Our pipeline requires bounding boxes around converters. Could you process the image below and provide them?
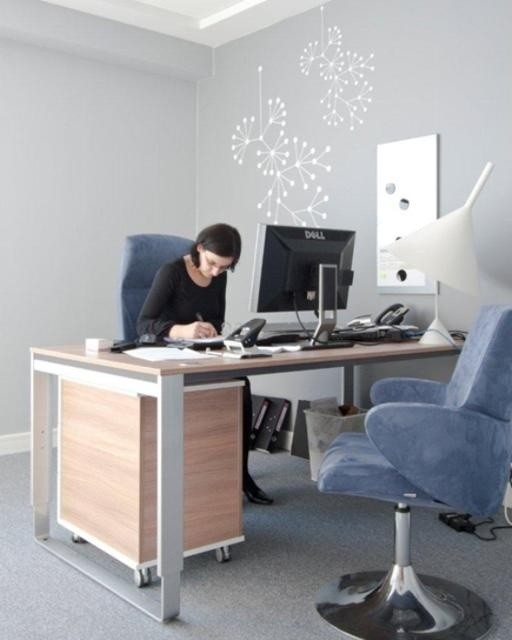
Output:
[438,512,476,531]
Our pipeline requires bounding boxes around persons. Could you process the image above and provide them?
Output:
[136,224,274,504]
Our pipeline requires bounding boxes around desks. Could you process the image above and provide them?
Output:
[28,331,466,623]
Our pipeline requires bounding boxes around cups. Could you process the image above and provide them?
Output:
[138,333,156,346]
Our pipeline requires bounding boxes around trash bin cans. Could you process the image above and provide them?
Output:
[303,404,370,482]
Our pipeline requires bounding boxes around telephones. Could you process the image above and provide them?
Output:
[375,304,410,326]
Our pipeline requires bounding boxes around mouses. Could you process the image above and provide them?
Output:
[140,333,158,346]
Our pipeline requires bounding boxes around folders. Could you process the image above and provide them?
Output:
[248,395,271,450]
[255,397,290,455]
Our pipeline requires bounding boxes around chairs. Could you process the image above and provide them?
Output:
[117,233,200,346]
[315,306,512,640]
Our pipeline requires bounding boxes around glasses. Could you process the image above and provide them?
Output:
[204,249,233,272]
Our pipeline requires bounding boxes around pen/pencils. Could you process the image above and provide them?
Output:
[196,312,204,322]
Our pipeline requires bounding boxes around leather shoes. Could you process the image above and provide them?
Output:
[243,475,274,506]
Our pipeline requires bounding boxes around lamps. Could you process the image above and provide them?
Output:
[379,163,497,349]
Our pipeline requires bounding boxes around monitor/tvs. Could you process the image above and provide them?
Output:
[249,222,357,352]
